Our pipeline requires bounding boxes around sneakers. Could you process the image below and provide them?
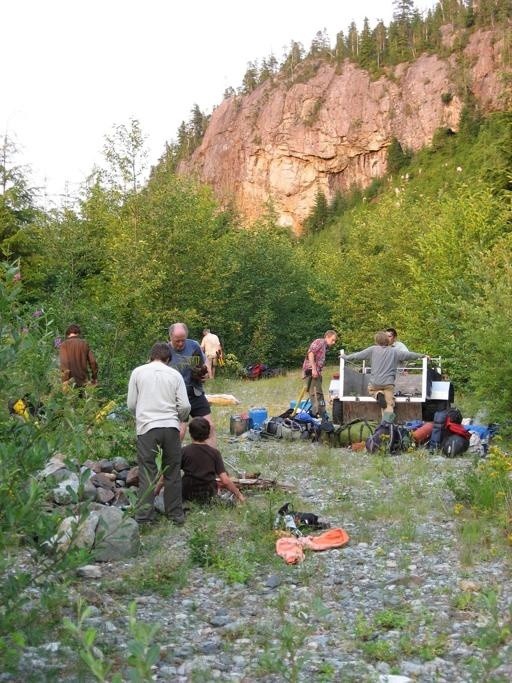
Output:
[376,391,387,408]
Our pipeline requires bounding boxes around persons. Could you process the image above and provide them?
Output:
[62,325,98,398]
[200,328,222,380]
[302,330,338,418]
[328,371,343,424]
[126,344,191,528]
[386,329,410,374]
[154,416,245,510]
[339,331,431,419]
[166,322,216,448]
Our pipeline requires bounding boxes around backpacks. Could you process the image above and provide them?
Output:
[337,416,378,446]
[403,406,472,457]
[265,409,337,445]
[366,421,401,455]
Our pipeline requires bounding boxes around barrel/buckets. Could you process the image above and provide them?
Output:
[249,406,267,429]
